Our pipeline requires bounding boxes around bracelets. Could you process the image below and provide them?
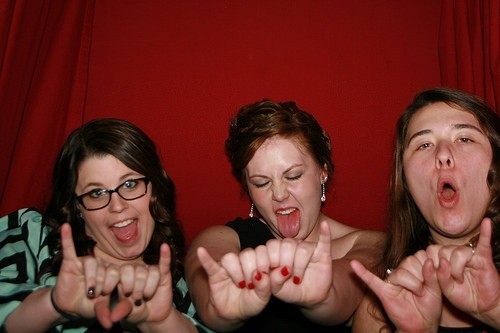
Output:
[50,285,88,323]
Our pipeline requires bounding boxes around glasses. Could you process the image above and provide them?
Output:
[73,173,154,211]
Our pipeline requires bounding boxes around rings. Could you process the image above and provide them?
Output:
[386,268,395,285]
[464,242,475,258]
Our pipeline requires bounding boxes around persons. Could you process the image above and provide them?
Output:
[349,87,500,333]
[183,98,389,333]
[0,118,211,333]
